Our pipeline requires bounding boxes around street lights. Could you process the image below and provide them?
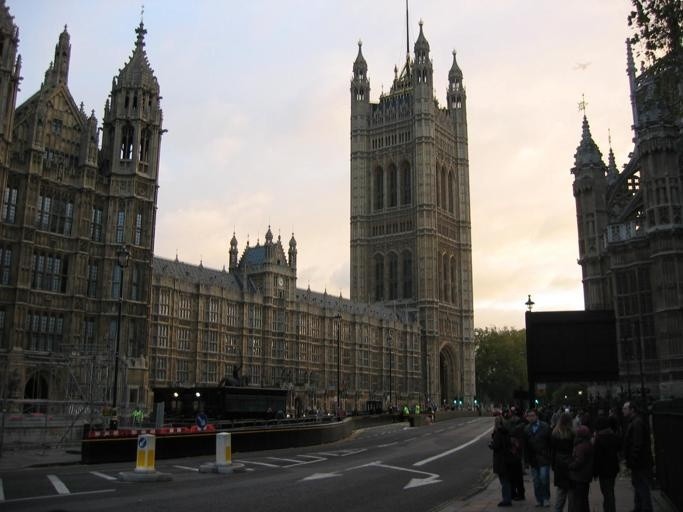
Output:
[334,313,342,413]
[387,333,394,406]
[110,244,134,430]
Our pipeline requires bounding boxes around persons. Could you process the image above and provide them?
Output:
[196,410,208,431]
[132,407,144,426]
[486,400,656,510]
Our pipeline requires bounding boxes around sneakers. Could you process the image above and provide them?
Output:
[497,493,551,508]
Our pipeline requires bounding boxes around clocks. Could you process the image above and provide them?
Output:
[277,276,284,287]
